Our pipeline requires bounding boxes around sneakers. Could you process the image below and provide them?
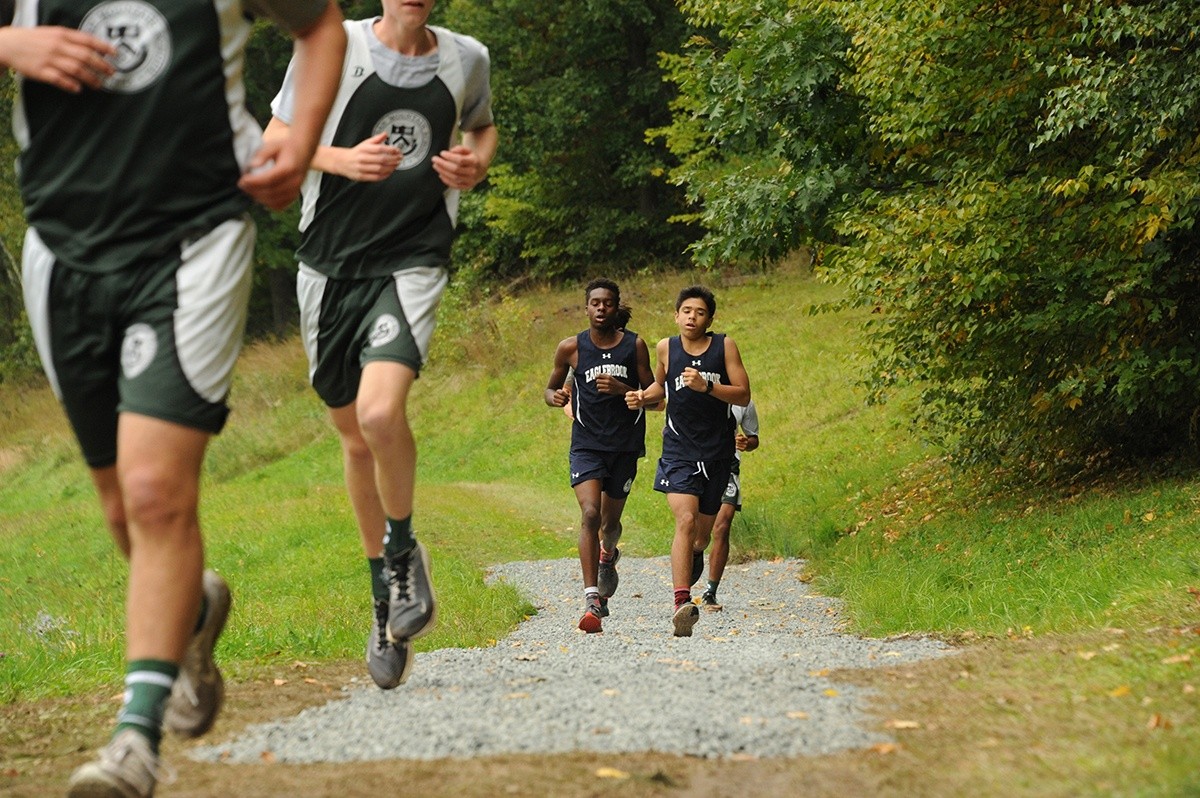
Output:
[577,604,603,633]
[699,592,722,612]
[381,540,437,642]
[68,727,158,796]
[162,568,231,739]
[692,549,703,586]
[672,601,699,637]
[597,547,621,598]
[367,595,414,689]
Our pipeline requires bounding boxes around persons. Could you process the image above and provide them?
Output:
[0,0,350,798]
[625,287,751,638]
[542,280,660,634]
[561,303,667,618]
[701,398,760,611]
[260,1,500,690]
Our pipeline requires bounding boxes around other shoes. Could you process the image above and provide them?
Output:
[600,596,610,617]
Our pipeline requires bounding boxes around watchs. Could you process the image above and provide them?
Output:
[704,380,713,394]
